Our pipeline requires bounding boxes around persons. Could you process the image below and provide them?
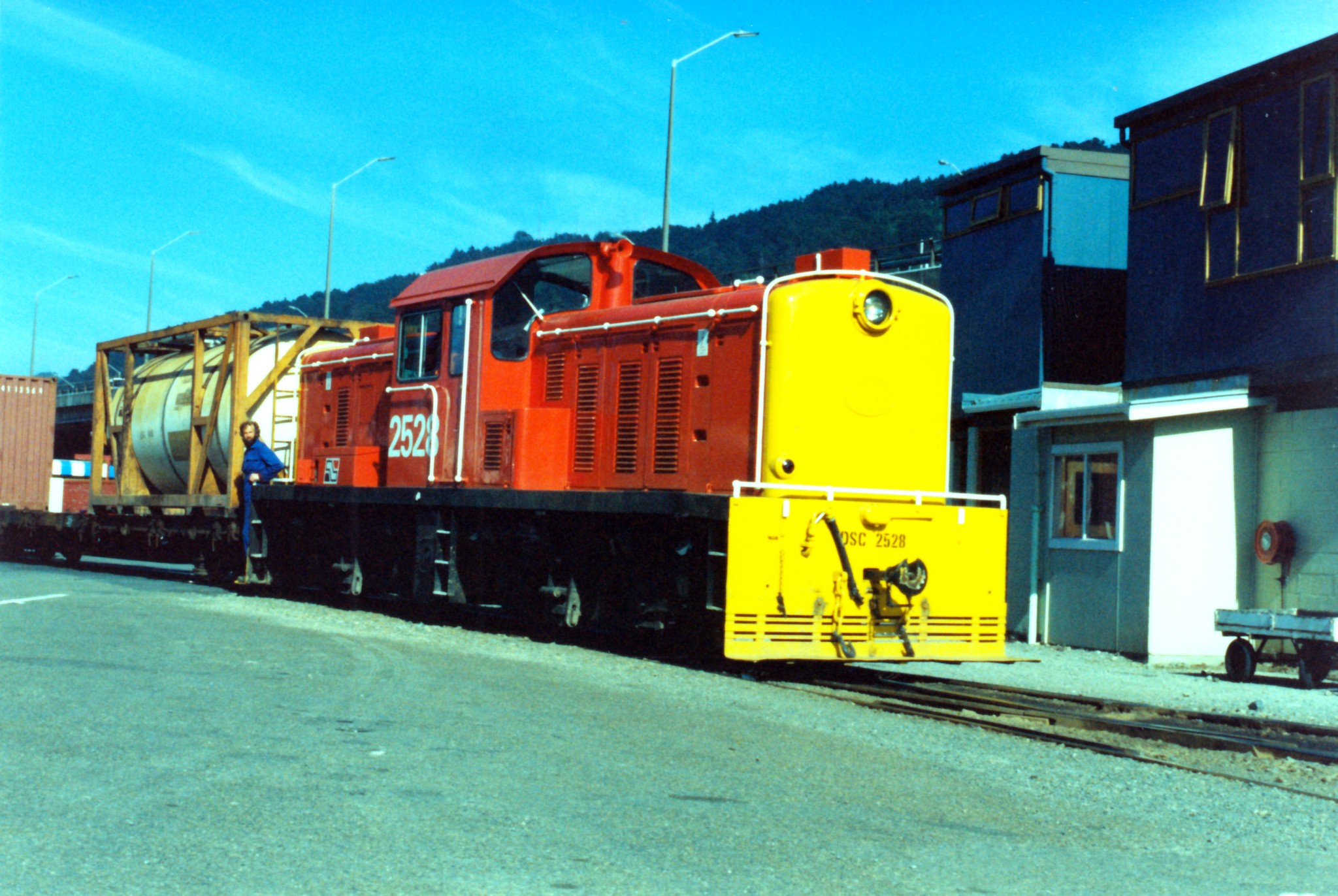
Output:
[235,421,285,584]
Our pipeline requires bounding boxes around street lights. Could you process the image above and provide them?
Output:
[325,153,395,321]
[664,32,766,254]
[149,233,195,330]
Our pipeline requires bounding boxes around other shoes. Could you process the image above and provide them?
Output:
[237,575,245,583]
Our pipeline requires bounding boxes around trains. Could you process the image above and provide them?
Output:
[0,238,1014,666]
